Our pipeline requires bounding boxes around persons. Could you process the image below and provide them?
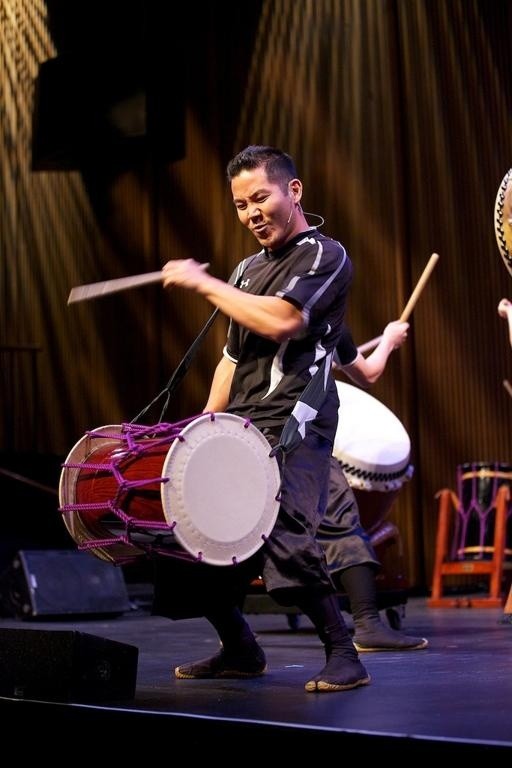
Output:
[313,316,429,655]
[162,142,372,692]
[497,298,512,350]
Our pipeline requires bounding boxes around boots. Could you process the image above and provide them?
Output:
[352,598,428,651]
[305,621,372,691]
[175,623,267,677]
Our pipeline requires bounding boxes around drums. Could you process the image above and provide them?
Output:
[454,460,510,560]
[332,380,413,537]
[57,411,282,569]
[495,165,512,275]
